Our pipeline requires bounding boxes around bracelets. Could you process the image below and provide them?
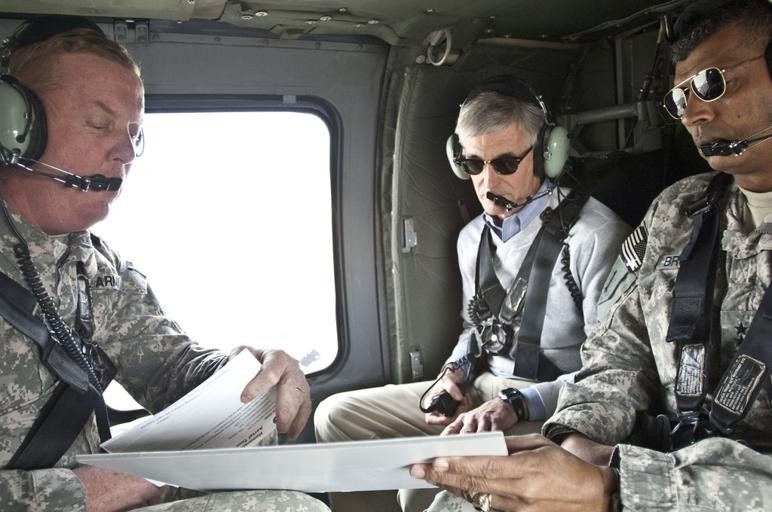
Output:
[607,462,623,512]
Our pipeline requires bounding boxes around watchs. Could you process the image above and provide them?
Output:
[498,386,525,423]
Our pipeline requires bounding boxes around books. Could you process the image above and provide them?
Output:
[97,347,279,489]
[73,428,510,495]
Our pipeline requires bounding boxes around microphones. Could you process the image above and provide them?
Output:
[699,133,771,157]
[16,160,122,193]
[485,181,558,211]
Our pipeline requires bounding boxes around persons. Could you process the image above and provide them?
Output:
[310,73,634,512]
[1,14,332,512]
[408,2,772,512]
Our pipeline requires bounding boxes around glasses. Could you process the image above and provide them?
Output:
[662,55,762,120]
[461,145,533,175]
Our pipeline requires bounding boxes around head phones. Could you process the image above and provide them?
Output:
[0,15,105,169]
[446,74,570,179]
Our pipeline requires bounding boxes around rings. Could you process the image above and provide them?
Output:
[295,386,305,393]
[471,492,492,512]
